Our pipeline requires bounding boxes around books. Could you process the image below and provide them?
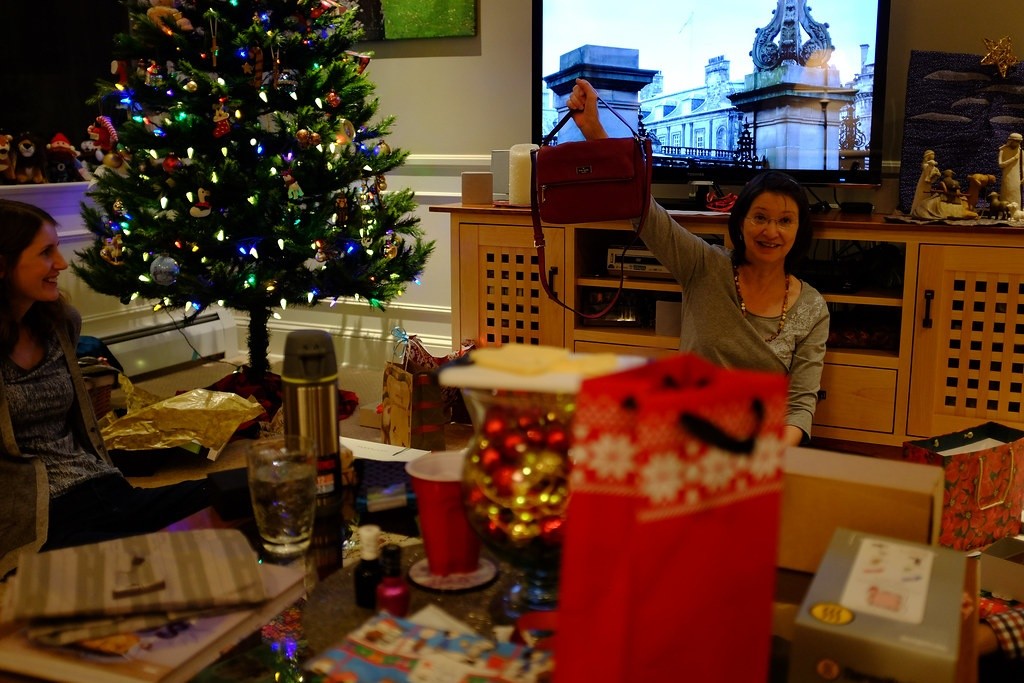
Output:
[0,529,305,683]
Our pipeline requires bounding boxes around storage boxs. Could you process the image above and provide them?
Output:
[788,521,981,678]
[755,446,955,576]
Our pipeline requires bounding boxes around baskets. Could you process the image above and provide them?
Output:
[85,375,114,420]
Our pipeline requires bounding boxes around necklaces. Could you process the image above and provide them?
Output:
[733,266,790,343]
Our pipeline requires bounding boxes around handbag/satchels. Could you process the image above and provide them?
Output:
[902,420,1024,552]
[531,97,652,318]
[381,338,447,454]
[552,351,787,683]
[405,334,478,413]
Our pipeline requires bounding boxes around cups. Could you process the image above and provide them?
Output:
[246,436,316,553]
[405,449,480,571]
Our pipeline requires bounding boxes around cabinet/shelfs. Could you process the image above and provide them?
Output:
[426,204,1018,443]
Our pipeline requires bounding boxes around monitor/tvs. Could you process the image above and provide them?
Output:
[532,0,891,211]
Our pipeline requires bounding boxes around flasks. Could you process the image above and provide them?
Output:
[282,328,340,470]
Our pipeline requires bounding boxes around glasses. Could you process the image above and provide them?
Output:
[745,214,800,229]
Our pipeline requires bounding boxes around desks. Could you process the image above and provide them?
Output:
[0,437,1024,683]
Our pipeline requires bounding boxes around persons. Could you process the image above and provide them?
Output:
[0,198,139,552]
[566,79,830,448]
[910,151,934,216]
[968,175,996,205]
[998,133,1024,209]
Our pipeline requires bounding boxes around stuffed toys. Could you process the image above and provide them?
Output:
[0,133,82,184]
